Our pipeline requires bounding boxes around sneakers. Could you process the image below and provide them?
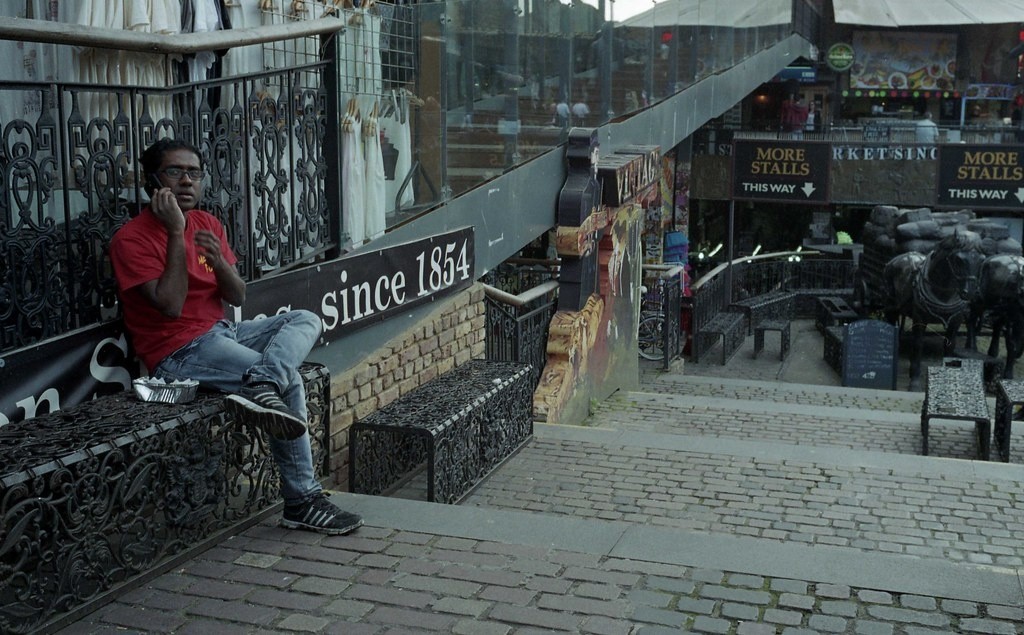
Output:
[224,386,308,441]
[282,491,365,535]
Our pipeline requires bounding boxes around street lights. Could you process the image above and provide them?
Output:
[566,2,576,129]
[438,13,456,196]
[505,6,523,167]
[607,0,617,119]
[650,0,659,103]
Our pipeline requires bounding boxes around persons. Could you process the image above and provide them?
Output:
[778,78,809,139]
[551,93,591,127]
[587,20,629,117]
[109,137,364,534]
[915,111,939,143]
[802,100,823,134]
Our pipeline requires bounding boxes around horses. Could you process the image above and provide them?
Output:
[881,228,1023,377]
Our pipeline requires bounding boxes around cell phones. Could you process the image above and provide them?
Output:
[144,174,162,198]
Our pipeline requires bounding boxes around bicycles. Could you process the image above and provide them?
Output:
[639,286,681,361]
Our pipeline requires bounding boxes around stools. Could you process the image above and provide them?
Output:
[824,326,846,374]
[994,379,1024,463]
[753,318,791,360]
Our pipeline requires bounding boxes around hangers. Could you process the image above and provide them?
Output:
[223,0,389,31]
[228,75,425,138]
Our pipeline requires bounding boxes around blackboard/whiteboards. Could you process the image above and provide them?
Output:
[841,318,900,390]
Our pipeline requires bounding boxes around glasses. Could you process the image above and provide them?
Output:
[156,168,206,182]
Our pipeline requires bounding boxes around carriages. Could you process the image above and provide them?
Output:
[854,205,1024,390]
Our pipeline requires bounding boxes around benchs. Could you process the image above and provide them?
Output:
[920,366,991,460]
[350,358,535,506]
[0,361,334,635]
[728,290,797,337]
[797,287,855,318]
[695,312,746,365]
